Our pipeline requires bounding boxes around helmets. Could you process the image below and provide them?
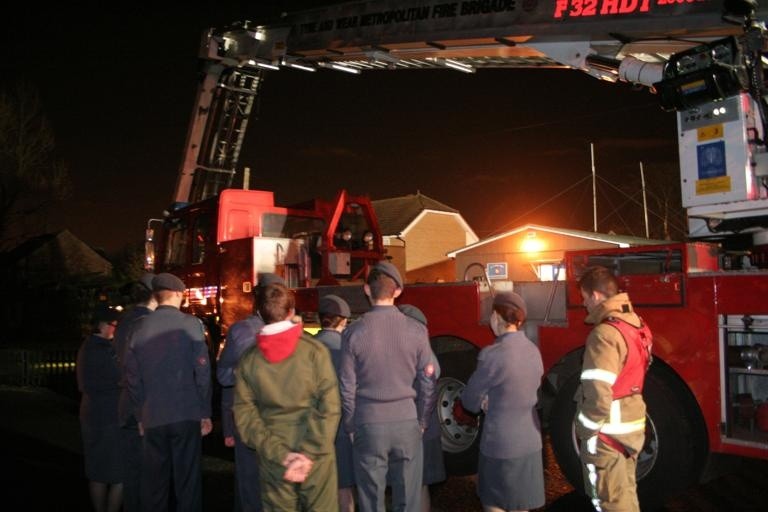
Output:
[398,303,427,323]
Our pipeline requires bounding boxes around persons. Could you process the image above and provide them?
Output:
[387,304,445,510]
[218,272,287,510]
[572,264,654,512]
[126,273,213,511]
[339,262,436,510]
[76,306,129,509]
[113,274,157,509]
[233,283,342,510]
[459,288,549,512]
[312,294,356,510]
[334,220,375,278]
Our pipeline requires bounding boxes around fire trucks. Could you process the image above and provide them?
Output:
[133,9,766,510]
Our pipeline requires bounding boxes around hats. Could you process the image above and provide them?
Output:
[317,294,351,317]
[252,272,286,293]
[493,290,527,312]
[140,273,186,291]
[370,261,403,287]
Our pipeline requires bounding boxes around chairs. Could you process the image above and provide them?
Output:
[736,393,762,434]
